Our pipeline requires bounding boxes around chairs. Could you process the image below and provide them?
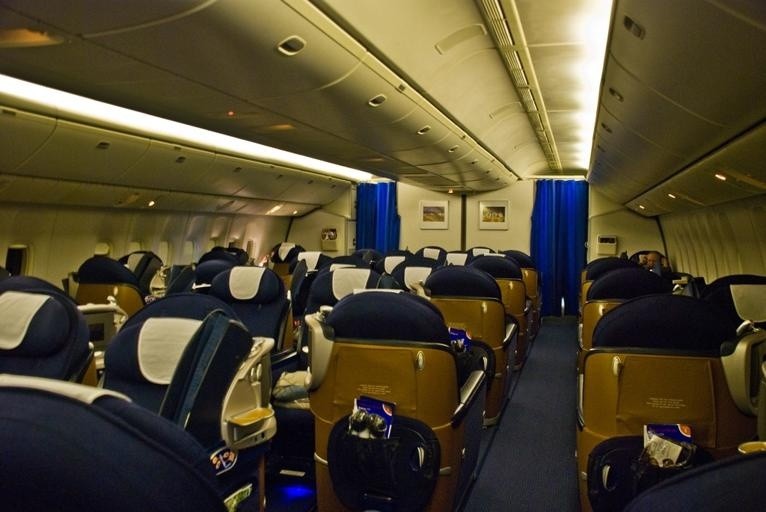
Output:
[568,243,766,511]
[0,244,547,511]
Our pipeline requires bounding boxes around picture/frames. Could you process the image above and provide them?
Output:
[418,199,450,230]
[479,199,510,231]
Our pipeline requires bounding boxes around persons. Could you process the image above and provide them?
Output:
[638,250,669,269]
[257,244,280,266]
